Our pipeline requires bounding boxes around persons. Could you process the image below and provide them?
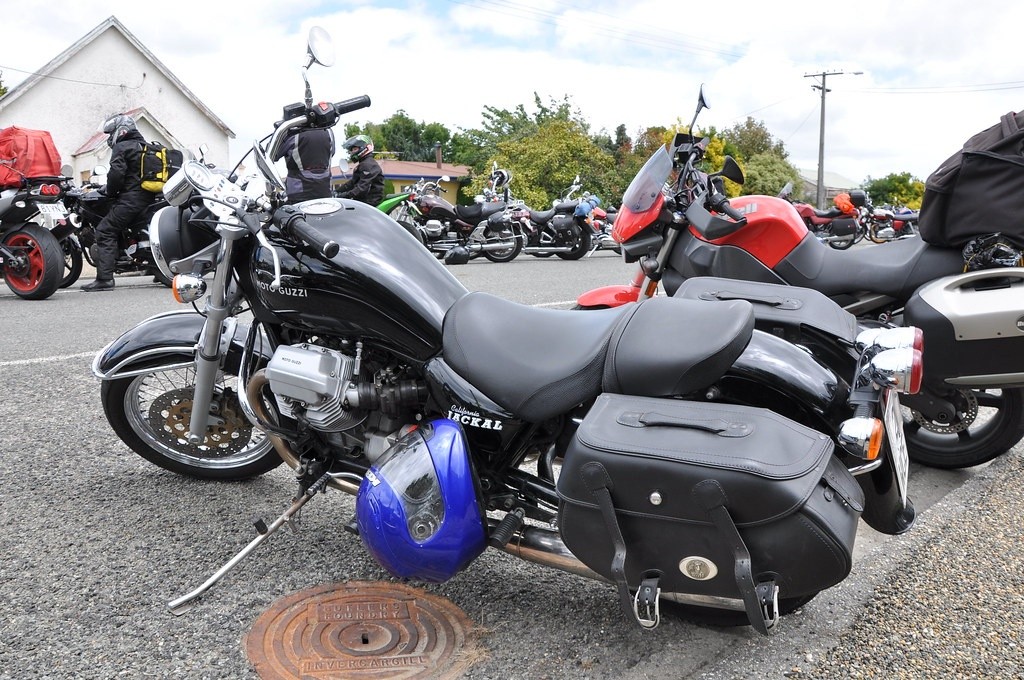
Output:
[332,134,387,207]
[272,127,335,205]
[80,112,155,293]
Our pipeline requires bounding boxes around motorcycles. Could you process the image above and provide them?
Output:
[0,175,74,300]
[90,22,925,637]
[474,161,598,260]
[570,82,1024,470]
[775,181,867,251]
[403,174,534,264]
[529,174,622,258]
[57,164,211,290]
[870,209,922,243]
[374,192,424,246]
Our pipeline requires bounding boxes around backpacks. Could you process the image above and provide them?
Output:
[917,110,1024,248]
[129,138,184,193]
[0,127,61,187]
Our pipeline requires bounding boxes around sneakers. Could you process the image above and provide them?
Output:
[80,278,115,292]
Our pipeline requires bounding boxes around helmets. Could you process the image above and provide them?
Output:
[104,113,137,148]
[490,169,512,189]
[355,418,489,585]
[342,134,374,163]
